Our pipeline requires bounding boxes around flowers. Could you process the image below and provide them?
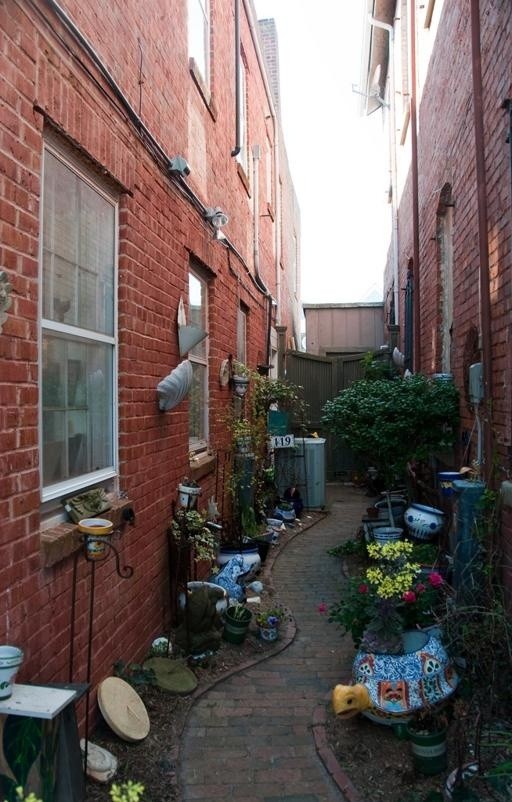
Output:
[317,539,445,649]
[253,600,292,627]
[364,538,423,655]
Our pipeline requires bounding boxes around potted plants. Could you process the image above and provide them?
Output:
[231,419,255,454]
[406,700,449,775]
[222,600,252,645]
[232,359,259,396]
[239,504,274,548]
[273,502,296,523]
[177,477,201,509]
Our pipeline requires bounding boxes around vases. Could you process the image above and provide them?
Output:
[373,471,465,544]
[333,630,460,740]
[256,621,280,642]
[390,603,418,632]
[216,544,262,582]
[78,518,113,560]
[0,644,24,700]
